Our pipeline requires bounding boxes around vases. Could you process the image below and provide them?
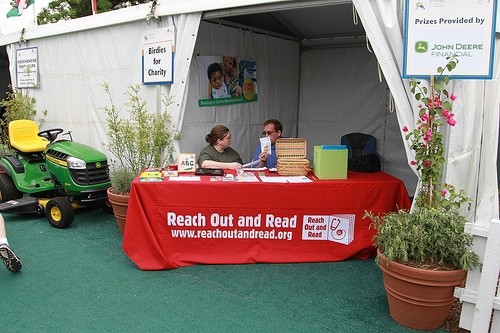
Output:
[374,244,467,331]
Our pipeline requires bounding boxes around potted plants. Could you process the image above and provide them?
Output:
[94,78,185,236]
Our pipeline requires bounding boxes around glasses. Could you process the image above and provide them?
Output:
[263,130,276,135]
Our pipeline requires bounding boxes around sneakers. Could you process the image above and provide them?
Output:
[0,243,23,272]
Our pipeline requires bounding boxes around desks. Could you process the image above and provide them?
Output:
[122,168,414,272]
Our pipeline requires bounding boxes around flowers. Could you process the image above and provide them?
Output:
[360,53,485,274]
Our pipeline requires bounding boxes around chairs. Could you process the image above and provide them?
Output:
[8,119,50,154]
[340,132,378,160]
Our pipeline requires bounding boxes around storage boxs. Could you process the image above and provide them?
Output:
[274,136,310,176]
[313,145,348,180]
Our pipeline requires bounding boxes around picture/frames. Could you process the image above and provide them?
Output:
[141,24,175,85]
[401,0,497,81]
[15,46,41,89]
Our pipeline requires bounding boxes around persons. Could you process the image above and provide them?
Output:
[197,123,248,172]
[0,214,23,273]
[207,62,233,98]
[251,118,290,170]
